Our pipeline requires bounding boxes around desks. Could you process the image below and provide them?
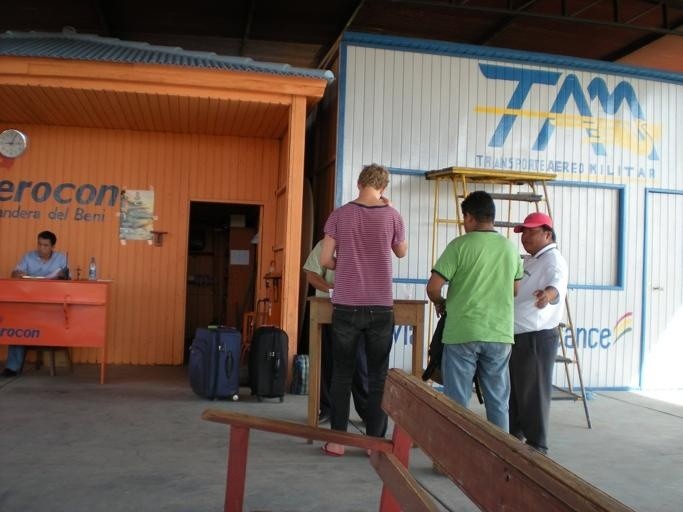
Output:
[0,276,112,386]
[303,296,429,445]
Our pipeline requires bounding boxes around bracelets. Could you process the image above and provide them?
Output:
[435,298,444,307]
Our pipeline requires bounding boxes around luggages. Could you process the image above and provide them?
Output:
[248,299,288,402]
[188,321,242,402]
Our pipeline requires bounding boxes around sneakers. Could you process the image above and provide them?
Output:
[319,411,331,424]
[3,367,17,378]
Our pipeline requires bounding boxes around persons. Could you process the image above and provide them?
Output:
[319,163,407,456]
[509,212,569,457]
[0,231,68,379]
[301,238,338,425]
[425,191,525,476]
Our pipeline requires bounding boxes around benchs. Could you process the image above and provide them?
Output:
[198,367,632,511]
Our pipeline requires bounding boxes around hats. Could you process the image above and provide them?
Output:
[514,213,553,233]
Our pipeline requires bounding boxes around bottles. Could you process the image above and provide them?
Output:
[88,257,96,281]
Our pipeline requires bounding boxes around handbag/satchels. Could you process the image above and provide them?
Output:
[422,311,447,386]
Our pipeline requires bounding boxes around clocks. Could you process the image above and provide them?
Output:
[0,129,27,159]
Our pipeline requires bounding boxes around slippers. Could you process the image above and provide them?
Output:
[321,443,344,456]
